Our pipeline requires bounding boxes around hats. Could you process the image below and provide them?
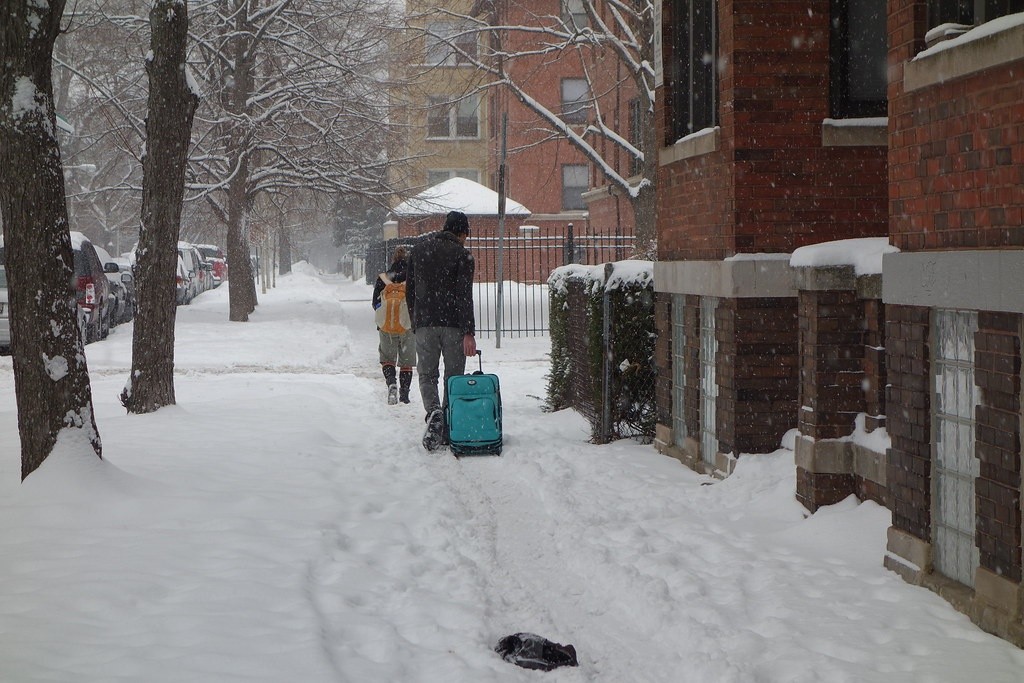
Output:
[444,211,469,235]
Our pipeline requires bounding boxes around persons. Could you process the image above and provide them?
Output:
[405,211,476,452]
[372,246,416,406]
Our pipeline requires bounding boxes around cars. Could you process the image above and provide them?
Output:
[0,231,260,356]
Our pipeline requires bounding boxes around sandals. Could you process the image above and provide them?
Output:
[387,384,399,405]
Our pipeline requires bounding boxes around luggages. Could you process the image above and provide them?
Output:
[444,349,502,457]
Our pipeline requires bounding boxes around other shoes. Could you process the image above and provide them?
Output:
[422,410,445,452]
[399,386,410,404]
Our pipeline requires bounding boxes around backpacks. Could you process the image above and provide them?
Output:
[374,272,412,335]
